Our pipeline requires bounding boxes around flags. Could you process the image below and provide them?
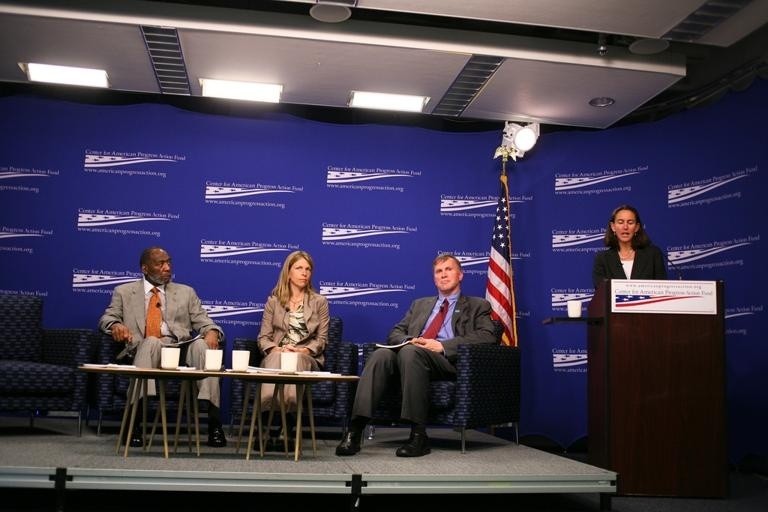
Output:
[486,180,517,348]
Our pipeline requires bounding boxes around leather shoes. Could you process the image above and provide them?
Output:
[275,438,295,452]
[396,431,431,457]
[336,431,362,456]
[253,437,273,452]
[207,426,227,447]
[122,425,142,447]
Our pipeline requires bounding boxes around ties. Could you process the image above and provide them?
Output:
[145,286,162,338]
[422,298,449,340]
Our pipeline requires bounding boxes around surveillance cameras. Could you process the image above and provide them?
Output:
[597,45,609,57]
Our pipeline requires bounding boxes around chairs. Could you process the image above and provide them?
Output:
[226,313,362,445]
[361,315,528,456]
[0,285,95,437]
[93,325,224,442]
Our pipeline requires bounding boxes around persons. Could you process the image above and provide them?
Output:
[593,206,668,288]
[97,247,227,448]
[335,254,496,457]
[255,249,331,454]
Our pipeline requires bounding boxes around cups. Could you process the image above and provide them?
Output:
[230,349,251,371]
[567,301,582,318]
[204,348,224,370]
[281,350,299,372]
[160,346,182,369]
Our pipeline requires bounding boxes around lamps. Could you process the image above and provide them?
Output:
[502,118,541,162]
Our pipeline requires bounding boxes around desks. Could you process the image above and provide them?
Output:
[76,362,222,460]
[223,367,360,462]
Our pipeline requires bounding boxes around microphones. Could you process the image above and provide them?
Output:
[648,242,687,280]
